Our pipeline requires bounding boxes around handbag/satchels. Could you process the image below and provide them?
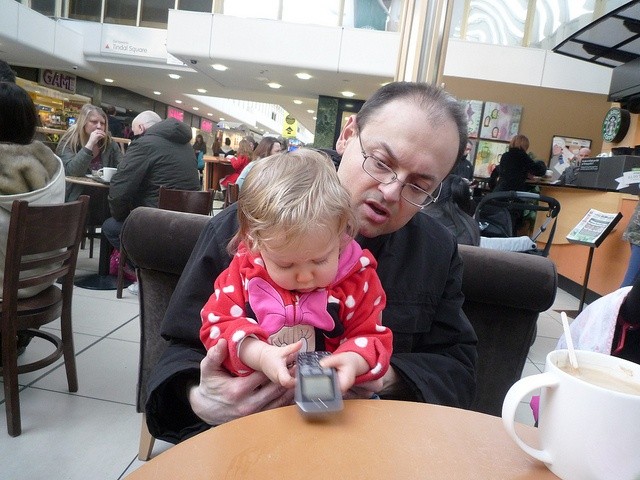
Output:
[197,150,205,169]
[110,248,137,282]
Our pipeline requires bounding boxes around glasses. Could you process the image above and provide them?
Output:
[357,125,442,208]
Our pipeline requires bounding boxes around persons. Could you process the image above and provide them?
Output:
[447,138,475,180]
[0,78,68,372]
[55,100,125,201]
[210,135,220,156]
[217,137,234,157]
[618,201,639,287]
[107,106,123,138]
[556,146,593,185]
[232,136,280,191]
[193,134,207,156]
[195,144,396,391]
[139,80,479,449]
[101,109,201,270]
[489,132,547,234]
[219,139,249,190]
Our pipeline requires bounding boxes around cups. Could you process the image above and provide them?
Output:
[501,349,640,480]
[219,153,225,159]
[97,166,117,183]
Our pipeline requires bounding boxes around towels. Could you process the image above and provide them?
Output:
[554,285,632,356]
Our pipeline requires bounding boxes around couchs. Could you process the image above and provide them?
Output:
[120,207,558,461]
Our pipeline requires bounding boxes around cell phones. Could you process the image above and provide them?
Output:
[293,350,343,415]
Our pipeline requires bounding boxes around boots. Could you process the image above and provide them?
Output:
[222,189,228,208]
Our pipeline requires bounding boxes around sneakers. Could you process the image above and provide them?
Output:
[128,281,139,296]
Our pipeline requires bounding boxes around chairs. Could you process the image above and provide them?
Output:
[116,185,214,300]
[472,191,560,258]
[224,182,239,206]
[0,195,90,437]
[81,224,103,258]
